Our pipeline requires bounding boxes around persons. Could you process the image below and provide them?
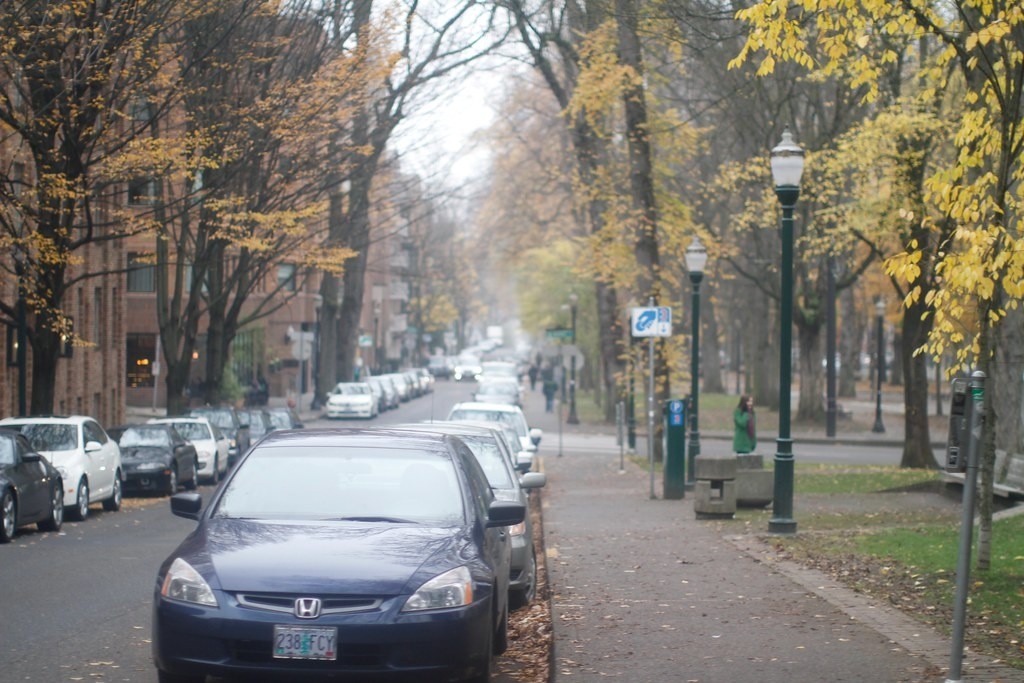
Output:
[536,351,543,367]
[541,364,553,393]
[529,363,539,389]
[196,377,270,407]
[734,394,757,454]
[542,376,559,413]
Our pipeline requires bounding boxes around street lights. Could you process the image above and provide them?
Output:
[767,120,806,534]
[681,235,718,477]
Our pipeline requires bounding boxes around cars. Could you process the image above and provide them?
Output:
[104,421,199,497]
[143,416,230,484]
[2,413,127,521]
[155,422,528,683]
[0,427,66,543]
[368,321,548,607]
[186,402,304,478]
[325,381,379,419]
